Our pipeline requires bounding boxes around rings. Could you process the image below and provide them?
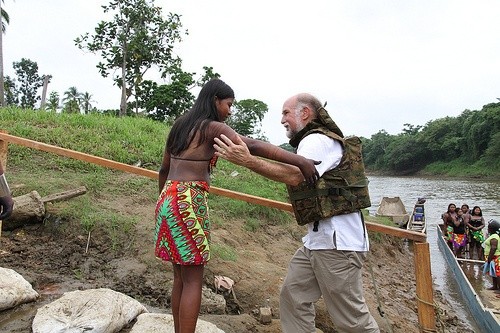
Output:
[223,151,225,154]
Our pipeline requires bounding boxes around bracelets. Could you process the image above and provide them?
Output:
[0,173,11,199]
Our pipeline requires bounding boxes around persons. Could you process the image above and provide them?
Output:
[154,78,322,333]
[483,218,500,300]
[441,203,486,265]
[0,159,14,220]
[211,91,381,333]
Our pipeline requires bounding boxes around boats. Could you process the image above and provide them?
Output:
[375,196,410,228]
[436,220,500,333]
[406,197,426,234]
